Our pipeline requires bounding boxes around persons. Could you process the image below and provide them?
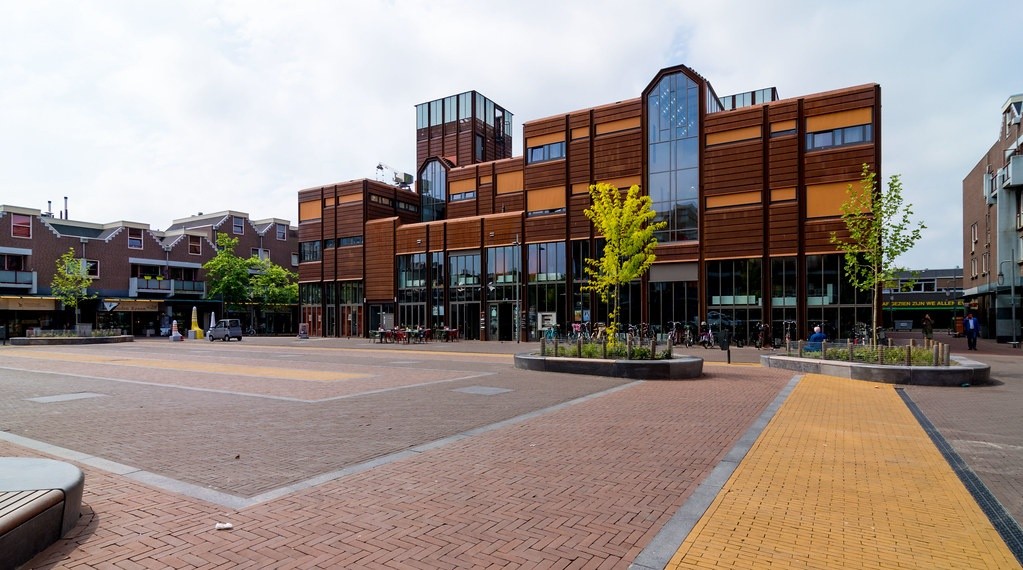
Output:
[922,314,934,340]
[378,322,449,343]
[962,312,981,351]
[810,327,826,342]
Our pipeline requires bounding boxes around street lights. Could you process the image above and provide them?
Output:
[945,269,957,334]
[889,288,895,331]
[997,249,1017,342]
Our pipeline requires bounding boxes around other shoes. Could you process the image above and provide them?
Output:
[968,346,977,350]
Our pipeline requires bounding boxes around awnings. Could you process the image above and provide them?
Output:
[0,294,64,310]
[97,297,223,312]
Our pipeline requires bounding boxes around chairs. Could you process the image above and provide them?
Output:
[368,327,459,343]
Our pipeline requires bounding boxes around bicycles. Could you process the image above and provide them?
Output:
[683,324,694,349]
[567,321,660,345]
[545,323,560,344]
[756,322,769,349]
[782,321,797,347]
[667,321,682,346]
[701,321,716,348]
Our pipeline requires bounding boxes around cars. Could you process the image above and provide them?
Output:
[208,318,242,342]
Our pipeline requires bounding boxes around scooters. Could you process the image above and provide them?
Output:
[812,322,825,336]
[852,322,889,346]
